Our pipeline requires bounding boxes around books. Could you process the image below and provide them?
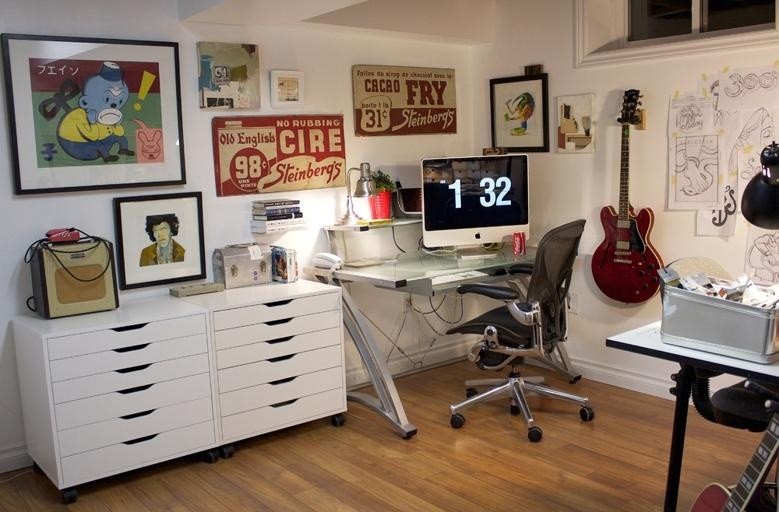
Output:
[250,199,306,233]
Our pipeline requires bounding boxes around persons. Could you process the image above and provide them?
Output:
[140,214,185,267]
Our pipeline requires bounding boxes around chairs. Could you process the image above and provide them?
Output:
[449,219,595,443]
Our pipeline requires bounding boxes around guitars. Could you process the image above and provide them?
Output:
[591,89,664,303]
[689,407,779,511]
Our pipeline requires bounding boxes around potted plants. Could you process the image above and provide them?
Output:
[368,169,392,220]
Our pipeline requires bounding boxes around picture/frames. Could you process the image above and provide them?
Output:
[270,70,304,109]
[196,41,261,111]
[489,72,550,154]
[112,191,207,290]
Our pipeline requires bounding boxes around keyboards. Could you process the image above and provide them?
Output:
[432,270,488,287]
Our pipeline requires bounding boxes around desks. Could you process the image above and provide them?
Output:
[604,316,779,512]
[303,218,538,437]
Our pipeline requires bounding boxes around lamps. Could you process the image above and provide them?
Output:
[333,162,378,226]
[741,142,779,230]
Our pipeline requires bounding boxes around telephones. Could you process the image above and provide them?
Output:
[312,253,343,285]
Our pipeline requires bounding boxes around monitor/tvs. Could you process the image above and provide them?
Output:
[421,154,530,261]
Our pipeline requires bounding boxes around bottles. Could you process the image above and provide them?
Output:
[514,231,526,256]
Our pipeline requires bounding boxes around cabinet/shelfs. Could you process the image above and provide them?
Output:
[1,32,187,196]
[11,294,220,491]
[179,279,347,446]
